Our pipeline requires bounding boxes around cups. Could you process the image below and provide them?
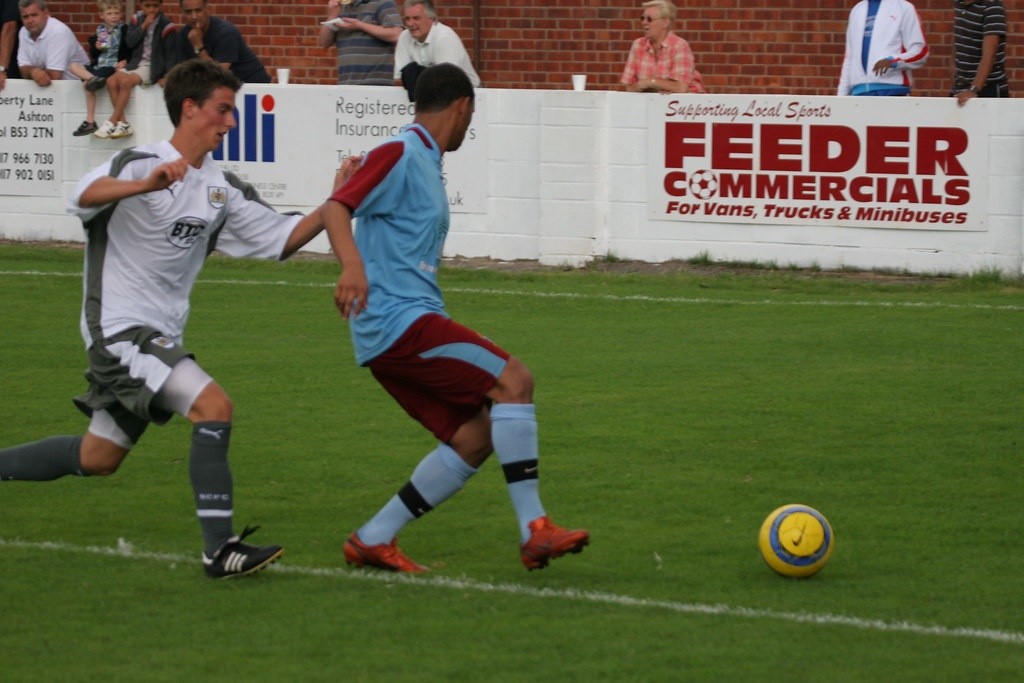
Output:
[572,74,587,91]
[276,68,290,84]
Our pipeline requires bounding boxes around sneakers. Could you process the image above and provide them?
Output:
[86,77,105,91]
[343,531,430,573]
[73,121,98,136]
[110,121,133,136]
[96,120,116,138]
[520,516,589,570]
[201,524,284,582]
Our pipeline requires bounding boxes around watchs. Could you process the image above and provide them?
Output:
[194,47,204,54]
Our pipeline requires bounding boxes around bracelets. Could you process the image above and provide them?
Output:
[970,85,979,95]
[652,79,655,85]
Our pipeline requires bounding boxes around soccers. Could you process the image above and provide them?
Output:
[758,505,834,578]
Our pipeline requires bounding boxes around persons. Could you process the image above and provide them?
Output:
[0,59,365,581]
[319,63,589,574]
[393,0,484,87]
[0,0,273,138]
[319,0,404,86]
[949,0,1010,108]
[836,0,930,96]
[621,0,707,94]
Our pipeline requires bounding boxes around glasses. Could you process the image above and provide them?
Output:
[640,16,665,22]
